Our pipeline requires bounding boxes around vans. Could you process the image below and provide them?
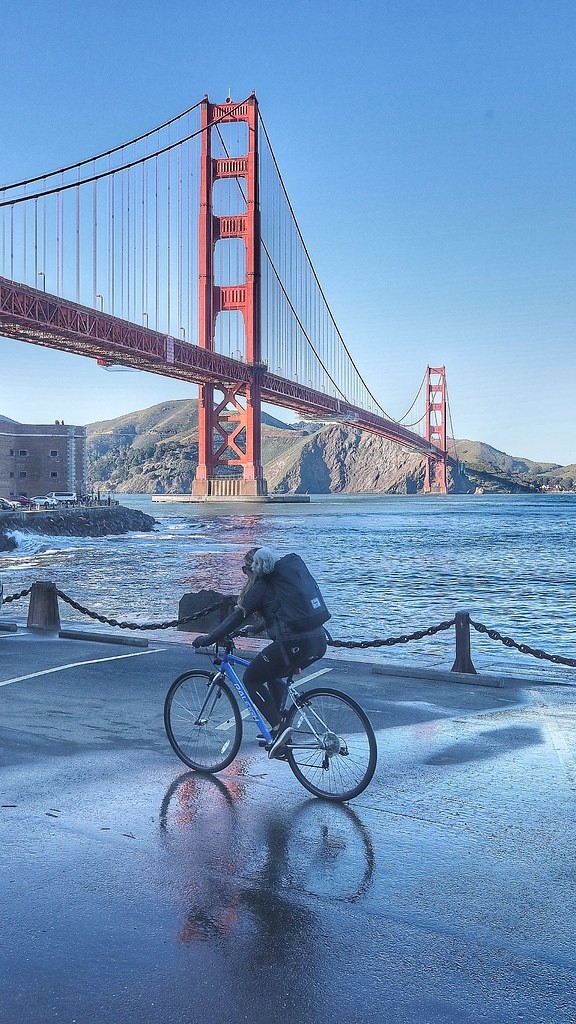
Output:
[46,491,77,503]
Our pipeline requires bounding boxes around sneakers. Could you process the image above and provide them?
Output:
[268,721,294,759]
[256,729,292,744]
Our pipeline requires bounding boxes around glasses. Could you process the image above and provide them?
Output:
[242,566,251,574]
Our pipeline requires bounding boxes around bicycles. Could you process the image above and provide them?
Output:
[163,628,378,802]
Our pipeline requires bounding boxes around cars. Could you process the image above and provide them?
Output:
[0,497,21,510]
[30,495,58,506]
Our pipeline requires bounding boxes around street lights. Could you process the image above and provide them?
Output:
[142,312,149,328]
[294,374,299,383]
[38,273,46,292]
[322,385,325,394]
[236,349,242,362]
[179,327,186,341]
[96,294,103,311]
[352,398,385,415]
[309,379,312,388]
[333,390,336,398]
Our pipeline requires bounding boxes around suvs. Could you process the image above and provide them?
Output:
[9,494,35,508]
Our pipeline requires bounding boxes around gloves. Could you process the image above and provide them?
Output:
[241,625,255,638]
[192,636,210,649]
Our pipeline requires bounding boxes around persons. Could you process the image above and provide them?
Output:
[190,546,327,759]
[79,493,97,505]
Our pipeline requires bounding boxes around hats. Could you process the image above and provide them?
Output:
[244,547,262,565]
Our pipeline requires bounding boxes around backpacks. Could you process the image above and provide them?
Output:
[258,553,332,631]
